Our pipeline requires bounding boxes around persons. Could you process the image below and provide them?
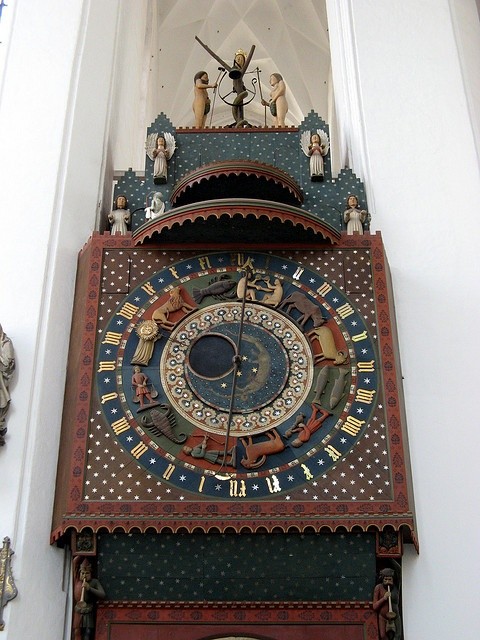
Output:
[308,133,325,181]
[152,135,169,184]
[183,432,234,467]
[373,558,402,640]
[132,366,155,408]
[291,403,329,448]
[71,555,105,640]
[144,192,165,221]
[192,70,218,129]
[108,195,131,235]
[344,195,368,235]
[261,73,289,128]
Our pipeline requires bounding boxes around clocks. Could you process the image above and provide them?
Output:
[51,230,419,552]
